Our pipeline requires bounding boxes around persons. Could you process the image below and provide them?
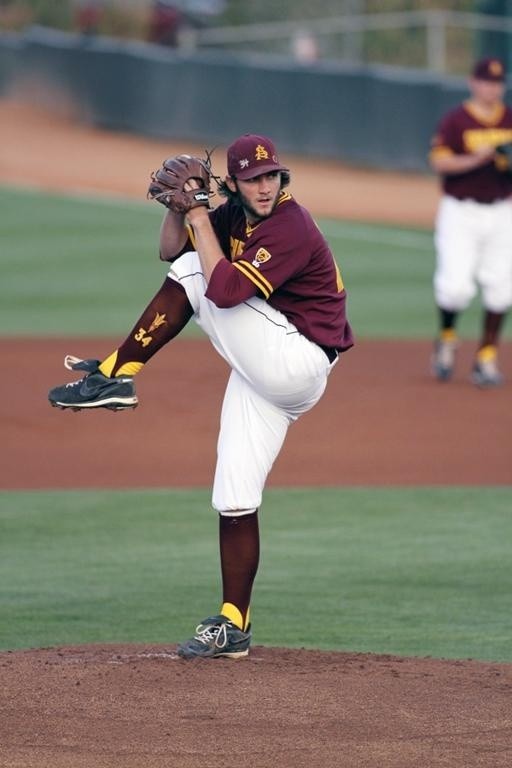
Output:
[421,57,511,389]
[46,132,356,662]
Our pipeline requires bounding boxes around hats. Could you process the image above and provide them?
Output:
[226,134,289,182]
[468,59,509,85]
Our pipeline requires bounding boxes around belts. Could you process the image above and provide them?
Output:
[314,339,337,366]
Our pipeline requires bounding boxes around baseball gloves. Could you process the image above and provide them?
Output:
[146,147,219,214]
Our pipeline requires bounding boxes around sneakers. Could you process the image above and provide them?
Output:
[430,334,502,389]
[178,615,252,658]
[47,355,140,411]
